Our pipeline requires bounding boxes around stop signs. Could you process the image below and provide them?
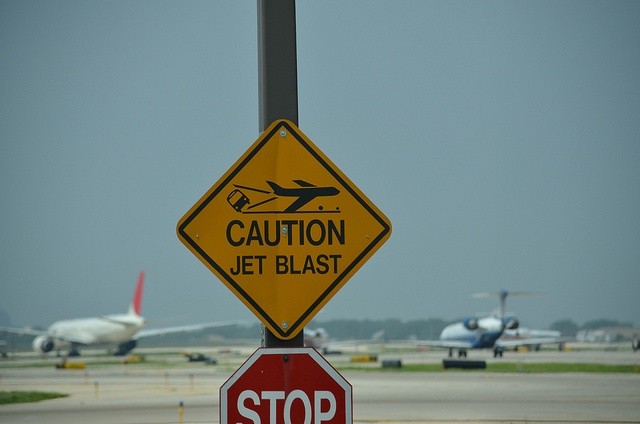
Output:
[218,346,355,424]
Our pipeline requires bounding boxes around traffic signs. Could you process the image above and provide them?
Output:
[173,118,392,340]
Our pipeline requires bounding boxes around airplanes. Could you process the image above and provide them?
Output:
[2,271,247,359]
[365,288,578,360]
